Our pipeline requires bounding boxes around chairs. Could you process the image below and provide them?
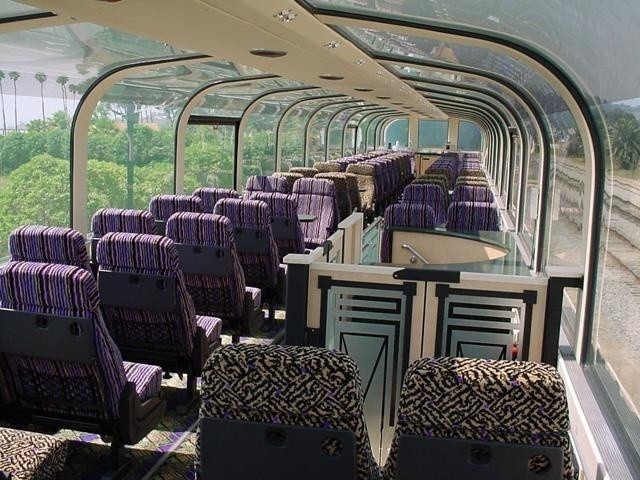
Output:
[165,211,265,343]
[380,357,580,480]
[194,343,381,478]
[247,161,382,190]
[293,191,381,240]
[90,208,160,261]
[150,195,204,234]
[237,191,310,254]
[382,150,498,264]
[331,151,381,161]
[212,198,286,320]
[10,224,96,284]
[192,187,238,213]
[0,262,167,470]
[96,231,223,398]
